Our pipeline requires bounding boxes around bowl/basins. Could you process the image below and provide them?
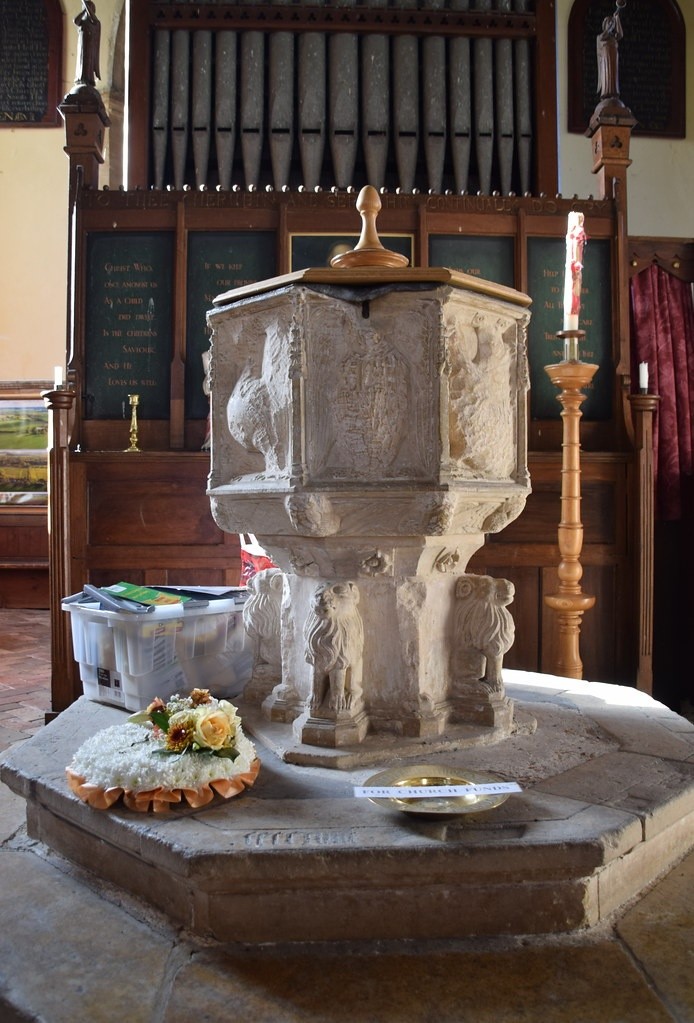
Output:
[361,764,508,822]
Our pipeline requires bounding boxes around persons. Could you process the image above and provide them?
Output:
[73,0,101,84]
[596,14,624,100]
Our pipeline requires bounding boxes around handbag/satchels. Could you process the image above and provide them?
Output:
[239,533,279,587]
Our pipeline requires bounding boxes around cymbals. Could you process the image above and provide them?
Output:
[363,764,524,822]
